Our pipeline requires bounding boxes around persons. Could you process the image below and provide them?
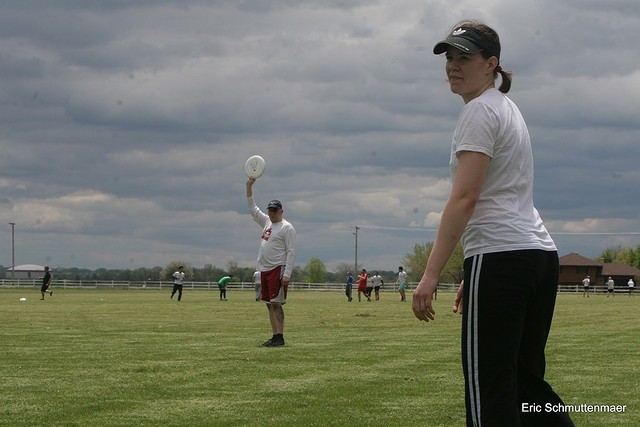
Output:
[395,266,408,302]
[345,272,355,301]
[40,266,53,300]
[373,272,384,300]
[607,277,615,298]
[582,275,591,297]
[627,278,635,297]
[253,267,262,301]
[431,283,439,300]
[245,176,297,348]
[170,266,185,301]
[411,18,575,426]
[366,273,374,301]
[358,269,371,302]
[217,274,232,301]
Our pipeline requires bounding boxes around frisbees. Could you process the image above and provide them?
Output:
[245,156,266,180]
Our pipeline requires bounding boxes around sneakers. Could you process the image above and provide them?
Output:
[262,339,274,346]
[265,338,285,347]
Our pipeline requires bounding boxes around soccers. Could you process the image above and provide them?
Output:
[17,294,29,306]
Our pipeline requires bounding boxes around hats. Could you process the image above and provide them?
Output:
[266,199,282,210]
[433,27,500,58]
